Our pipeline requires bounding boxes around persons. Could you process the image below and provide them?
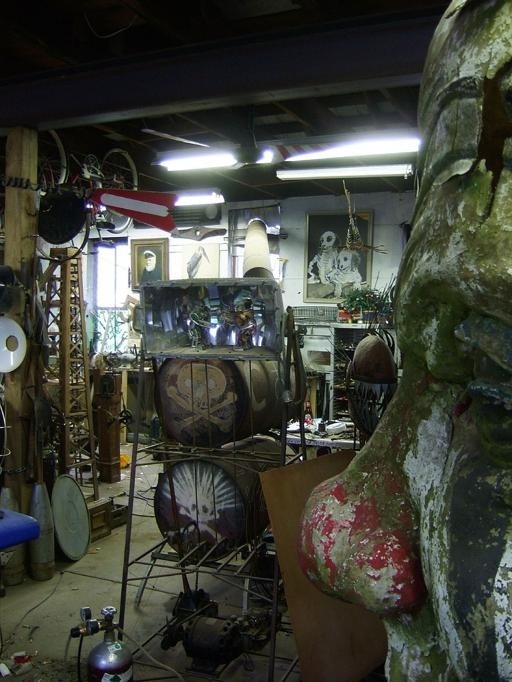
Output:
[139,249,160,285]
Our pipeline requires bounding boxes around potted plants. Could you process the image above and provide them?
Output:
[341,286,380,323]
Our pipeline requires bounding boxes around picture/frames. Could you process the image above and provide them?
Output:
[131,239,169,291]
[303,210,373,303]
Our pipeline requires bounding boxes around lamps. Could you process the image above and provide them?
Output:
[153,122,421,208]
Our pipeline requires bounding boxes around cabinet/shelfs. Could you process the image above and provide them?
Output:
[329,322,384,424]
